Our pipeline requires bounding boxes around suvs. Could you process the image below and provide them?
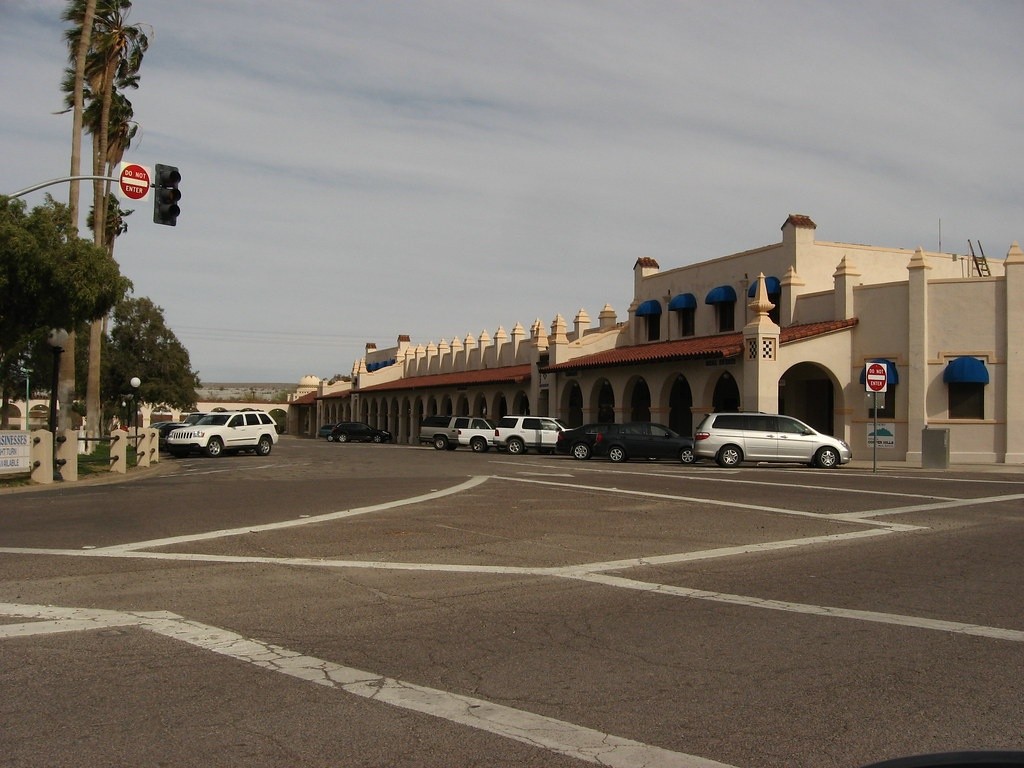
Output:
[149,413,208,452]
[419,414,495,450]
[690,411,853,468]
[166,410,280,459]
[492,415,579,454]
[329,422,393,443]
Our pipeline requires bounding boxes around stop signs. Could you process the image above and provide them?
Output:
[118,162,152,201]
[866,363,887,392]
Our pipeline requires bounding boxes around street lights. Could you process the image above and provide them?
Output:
[44,328,69,437]
[129,377,142,457]
[153,163,182,227]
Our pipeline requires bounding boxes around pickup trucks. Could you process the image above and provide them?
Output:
[457,428,496,453]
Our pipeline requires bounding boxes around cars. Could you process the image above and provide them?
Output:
[555,423,641,460]
[593,422,697,464]
[318,424,336,442]
